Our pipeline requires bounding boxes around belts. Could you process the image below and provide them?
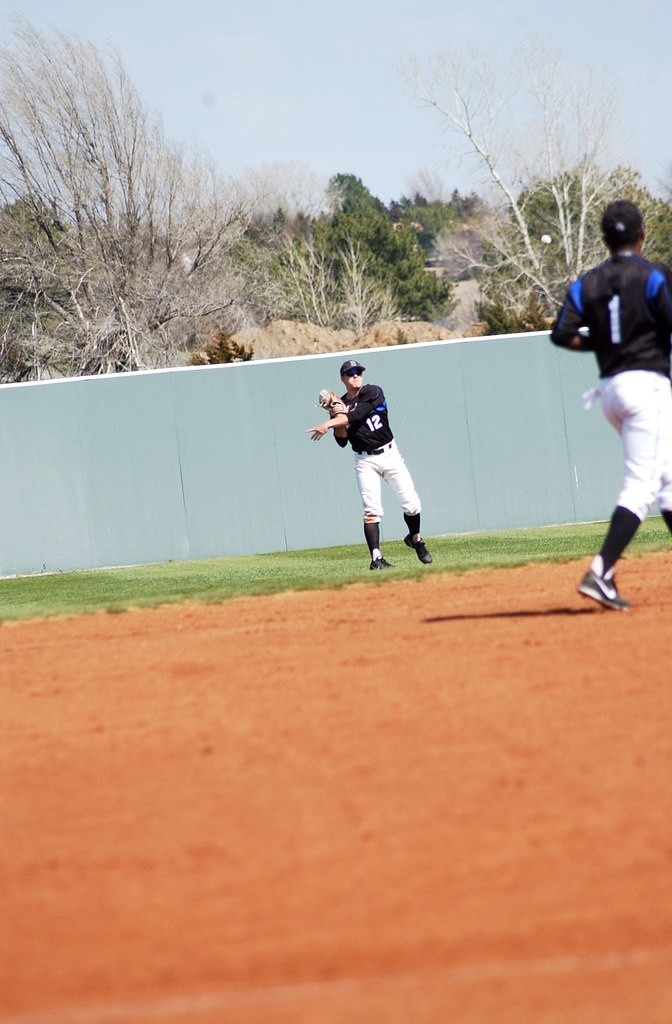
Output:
[357,444,391,455]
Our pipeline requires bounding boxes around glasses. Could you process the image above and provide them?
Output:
[342,368,363,375]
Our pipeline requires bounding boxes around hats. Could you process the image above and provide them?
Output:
[601,201,644,234]
[340,361,365,376]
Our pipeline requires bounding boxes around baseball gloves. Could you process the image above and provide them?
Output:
[323,392,349,416]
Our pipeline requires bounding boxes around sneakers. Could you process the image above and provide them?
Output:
[404,535,432,564]
[577,569,630,611]
[370,557,395,570]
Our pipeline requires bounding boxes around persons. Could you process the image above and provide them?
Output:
[305,360,432,570]
[550,201,672,612]
